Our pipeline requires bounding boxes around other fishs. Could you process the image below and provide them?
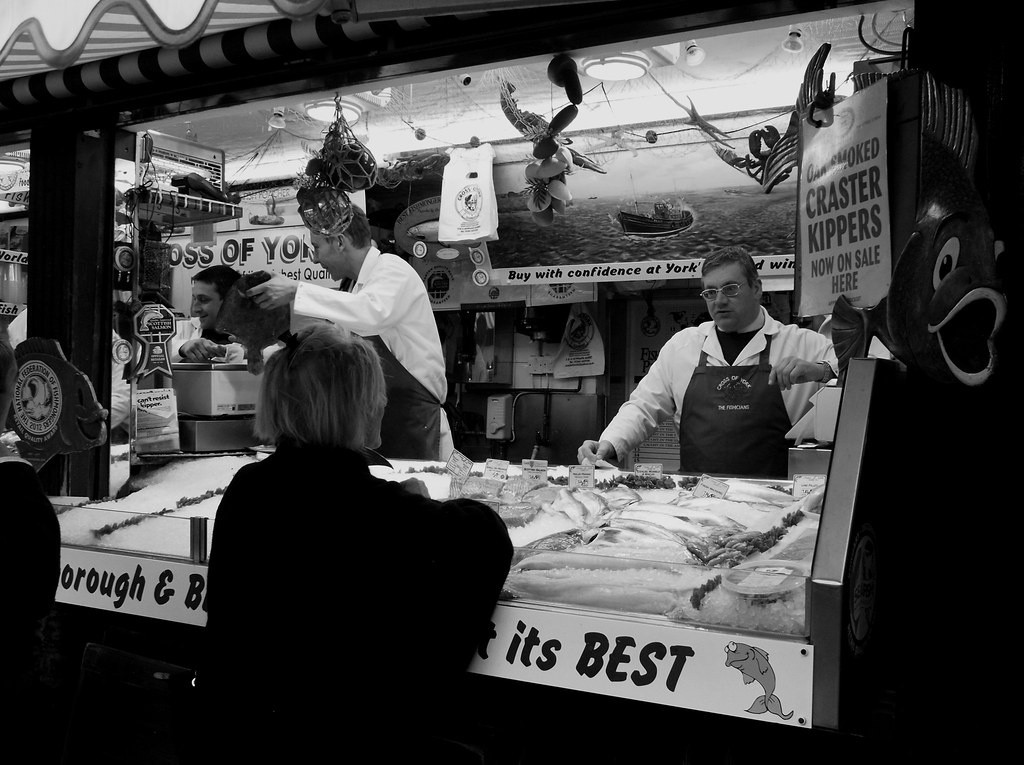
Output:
[210,270,290,376]
[458,474,826,566]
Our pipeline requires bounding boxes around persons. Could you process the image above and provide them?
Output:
[577,247,840,481]
[0,323,64,763]
[180,321,516,765]
[166,265,289,367]
[5,305,138,438]
[246,200,460,463]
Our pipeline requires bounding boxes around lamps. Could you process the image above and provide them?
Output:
[302,95,364,124]
[580,51,653,83]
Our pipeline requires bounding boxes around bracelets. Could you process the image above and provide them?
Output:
[816,361,832,383]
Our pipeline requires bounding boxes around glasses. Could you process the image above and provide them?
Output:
[699,281,751,300]
